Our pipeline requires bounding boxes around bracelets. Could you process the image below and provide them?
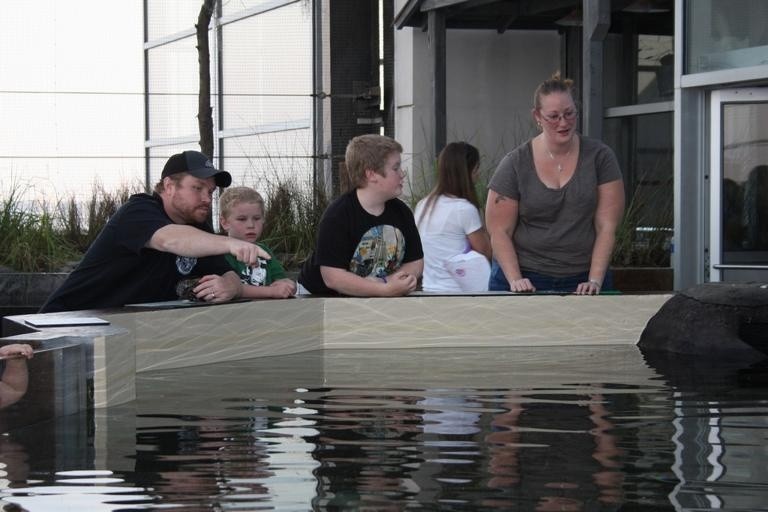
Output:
[587,279,603,289]
[380,276,388,284]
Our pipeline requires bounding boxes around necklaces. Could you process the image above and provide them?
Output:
[540,135,575,173]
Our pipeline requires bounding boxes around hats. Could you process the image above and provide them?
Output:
[162,151,231,187]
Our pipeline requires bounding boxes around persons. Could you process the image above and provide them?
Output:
[474,382,638,511]
[0,343,34,408]
[35,149,273,314]
[412,140,493,293]
[484,68,627,296]
[294,133,425,298]
[215,186,298,300]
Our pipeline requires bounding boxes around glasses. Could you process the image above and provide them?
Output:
[539,109,578,124]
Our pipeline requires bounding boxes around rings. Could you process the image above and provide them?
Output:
[208,286,214,293]
[212,293,215,298]
